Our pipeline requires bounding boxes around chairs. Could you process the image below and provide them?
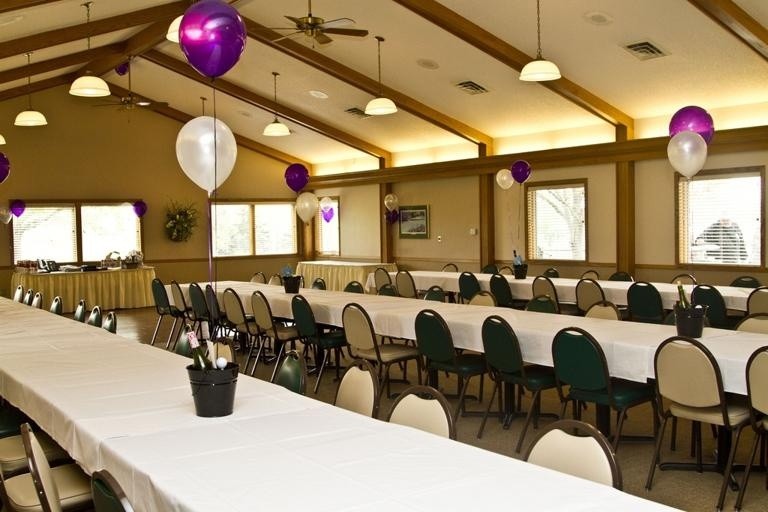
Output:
[0,259,767,512]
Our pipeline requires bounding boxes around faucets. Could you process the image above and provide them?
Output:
[104,249,119,265]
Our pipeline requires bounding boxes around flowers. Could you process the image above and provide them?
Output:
[165,198,199,242]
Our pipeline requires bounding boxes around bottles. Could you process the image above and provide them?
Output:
[676,280,690,308]
[186,331,210,372]
[513,249,518,265]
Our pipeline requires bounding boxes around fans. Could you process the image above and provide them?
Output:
[91,58,169,111]
[267,0,369,48]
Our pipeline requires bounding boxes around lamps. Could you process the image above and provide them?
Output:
[263,75,290,136]
[519,0,562,82]
[0,134,6,145]
[14,55,48,126]
[69,1,112,97]
[166,15,184,43]
[364,36,397,116]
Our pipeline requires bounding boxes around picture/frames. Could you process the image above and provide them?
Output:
[399,204,430,239]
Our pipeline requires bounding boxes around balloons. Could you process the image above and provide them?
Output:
[512,160,533,184]
[175,115,238,191]
[320,197,332,213]
[134,200,147,217]
[9,199,26,217]
[384,193,399,212]
[669,106,714,145]
[0,152,9,184]
[295,192,319,225]
[322,207,333,222]
[285,164,309,193]
[385,210,398,225]
[495,168,514,190]
[178,0,246,80]
[0,206,12,225]
[667,130,707,180]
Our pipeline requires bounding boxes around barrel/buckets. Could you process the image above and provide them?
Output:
[186,361,238,418]
[673,301,710,338]
[283,276,301,293]
[512,263,528,279]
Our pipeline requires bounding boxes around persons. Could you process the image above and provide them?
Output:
[695,219,748,264]
